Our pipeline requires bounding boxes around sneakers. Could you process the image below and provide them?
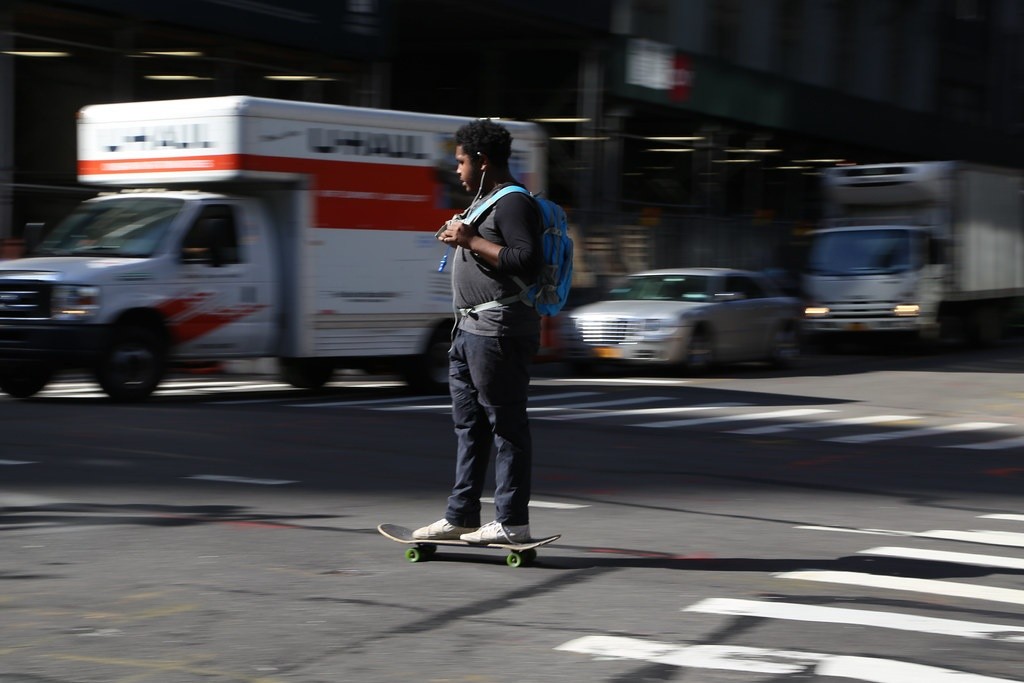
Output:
[459,521,531,544]
[412,518,480,540]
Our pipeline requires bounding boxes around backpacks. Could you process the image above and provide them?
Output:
[460,185,574,318]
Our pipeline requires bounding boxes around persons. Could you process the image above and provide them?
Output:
[410,115,541,544]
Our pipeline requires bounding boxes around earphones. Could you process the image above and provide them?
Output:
[477,152,482,156]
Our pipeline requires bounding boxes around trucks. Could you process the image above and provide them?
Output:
[0,93,550,407]
[793,158,1024,356]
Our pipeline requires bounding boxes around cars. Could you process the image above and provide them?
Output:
[547,263,802,377]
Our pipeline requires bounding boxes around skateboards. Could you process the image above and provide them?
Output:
[377,522,561,568]
